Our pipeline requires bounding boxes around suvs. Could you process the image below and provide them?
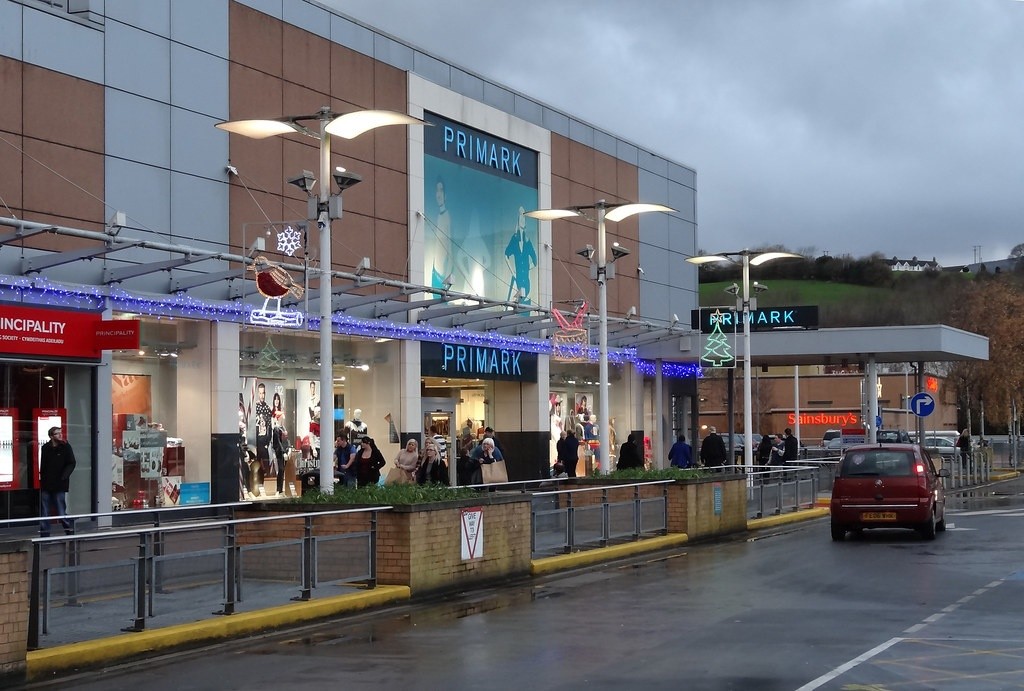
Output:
[877,429,912,444]
[831,443,951,542]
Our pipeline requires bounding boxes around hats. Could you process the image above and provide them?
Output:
[485,427,494,432]
[554,395,563,402]
[709,426,716,433]
[48,427,62,436]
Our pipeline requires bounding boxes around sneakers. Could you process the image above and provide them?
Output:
[773,473,783,479]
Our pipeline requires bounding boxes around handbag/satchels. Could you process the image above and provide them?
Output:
[553,459,565,473]
[481,458,508,484]
[384,463,402,486]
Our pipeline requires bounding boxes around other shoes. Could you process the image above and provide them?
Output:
[794,473,798,477]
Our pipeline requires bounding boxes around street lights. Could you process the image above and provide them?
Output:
[522,198,680,478]
[683,248,807,504]
[212,106,436,504]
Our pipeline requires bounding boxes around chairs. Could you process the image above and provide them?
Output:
[763,446,781,469]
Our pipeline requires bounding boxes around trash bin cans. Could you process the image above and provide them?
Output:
[296,467,348,499]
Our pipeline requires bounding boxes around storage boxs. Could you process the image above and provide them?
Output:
[141,431,167,448]
[158,476,182,508]
[122,430,141,462]
[180,481,211,506]
[127,413,148,430]
[140,478,157,507]
[164,446,185,477]
[140,448,163,477]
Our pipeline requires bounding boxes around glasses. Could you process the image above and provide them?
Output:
[53,431,62,435]
[426,448,435,452]
[555,402,561,407]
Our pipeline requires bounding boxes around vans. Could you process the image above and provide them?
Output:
[820,429,840,449]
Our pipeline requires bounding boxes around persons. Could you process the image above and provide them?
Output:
[238,411,256,499]
[741,428,798,484]
[256,383,286,477]
[585,415,602,473]
[394,425,506,488]
[273,414,291,494]
[345,409,367,453]
[309,406,321,468]
[333,434,386,489]
[504,206,537,317]
[432,180,453,305]
[700,426,727,467]
[557,430,579,477]
[956,429,972,475]
[608,416,616,471]
[40,427,76,537]
[617,434,638,471]
[308,382,319,406]
[668,435,695,469]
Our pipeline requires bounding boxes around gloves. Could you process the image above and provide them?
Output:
[764,463,769,469]
[772,446,778,452]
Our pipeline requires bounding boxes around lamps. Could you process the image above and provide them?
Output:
[574,301,585,315]
[513,287,525,302]
[625,306,636,320]
[158,346,170,357]
[247,237,267,257]
[106,210,127,235]
[669,313,680,328]
[356,258,371,275]
[170,347,183,358]
[442,273,456,290]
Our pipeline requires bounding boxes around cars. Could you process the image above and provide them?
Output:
[716,432,804,455]
[827,438,841,449]
[912,436,962,459]
